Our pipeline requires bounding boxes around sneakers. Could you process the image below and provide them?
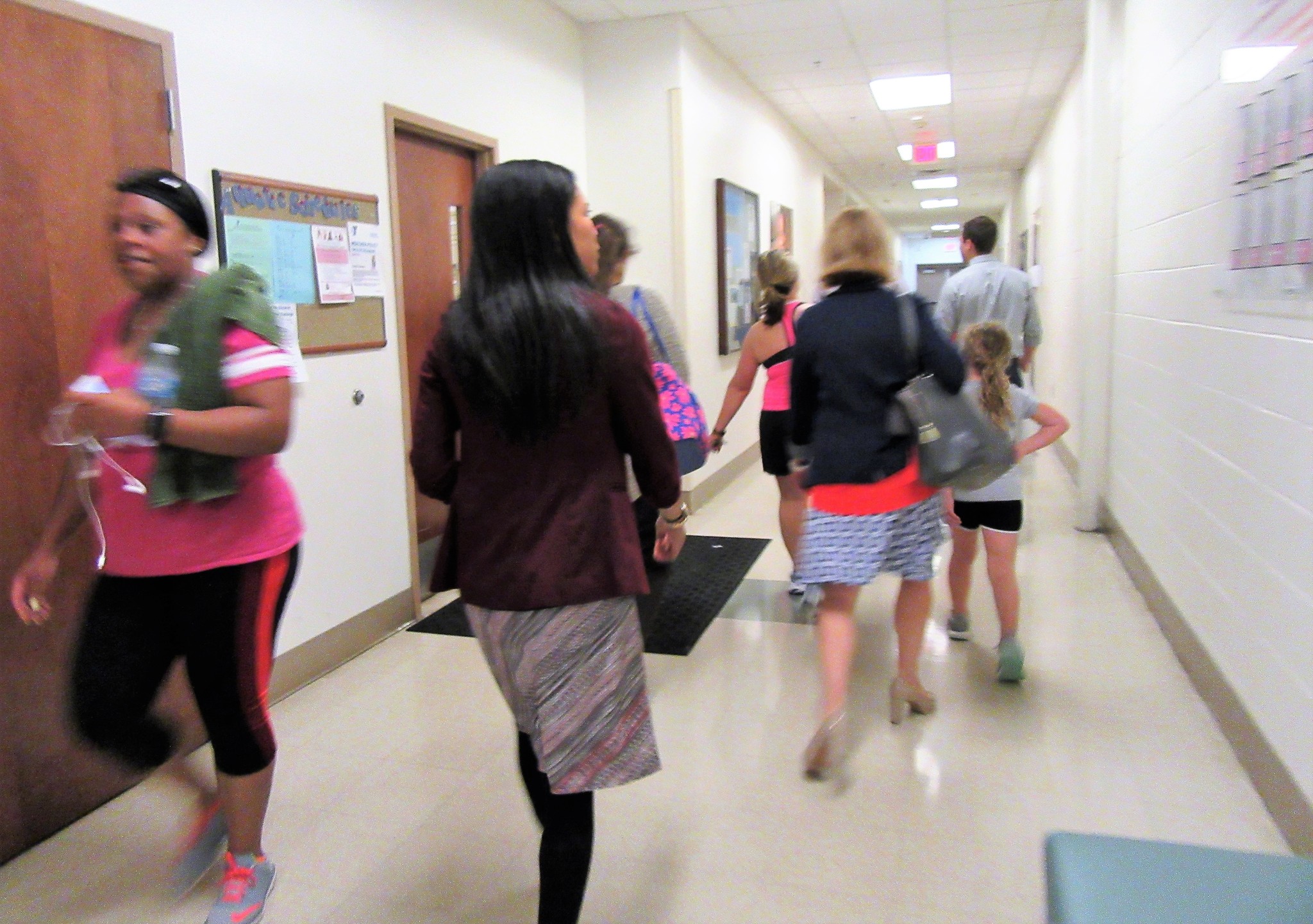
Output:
[168,796,231,900]
[205,851,276,924]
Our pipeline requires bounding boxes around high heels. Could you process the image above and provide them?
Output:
[805,705,850,782]
[889,675,936,725]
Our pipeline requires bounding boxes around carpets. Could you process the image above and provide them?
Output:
[407,531,772,661]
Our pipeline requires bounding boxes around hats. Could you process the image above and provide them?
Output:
[117,167,210,255]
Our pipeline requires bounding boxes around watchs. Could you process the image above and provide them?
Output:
[714,428,727,437]
[662,504,690,527]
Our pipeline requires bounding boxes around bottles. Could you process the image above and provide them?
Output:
[135,341,182,411]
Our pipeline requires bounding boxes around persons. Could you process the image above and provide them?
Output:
[12,168,302,924]
[406,160,687,924]
[589,208,1073,782]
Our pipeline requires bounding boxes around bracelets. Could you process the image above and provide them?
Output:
[143,406,169,441]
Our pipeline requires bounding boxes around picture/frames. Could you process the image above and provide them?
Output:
[714,175,765,358]
[771,200,795,256]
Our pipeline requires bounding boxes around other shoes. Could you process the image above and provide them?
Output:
[948,613,972,641]
[997,639,1023,684]
[787,571,810,595]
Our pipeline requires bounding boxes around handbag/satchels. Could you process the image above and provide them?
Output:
[631,288,708,475]
[899,291,1017,490]
